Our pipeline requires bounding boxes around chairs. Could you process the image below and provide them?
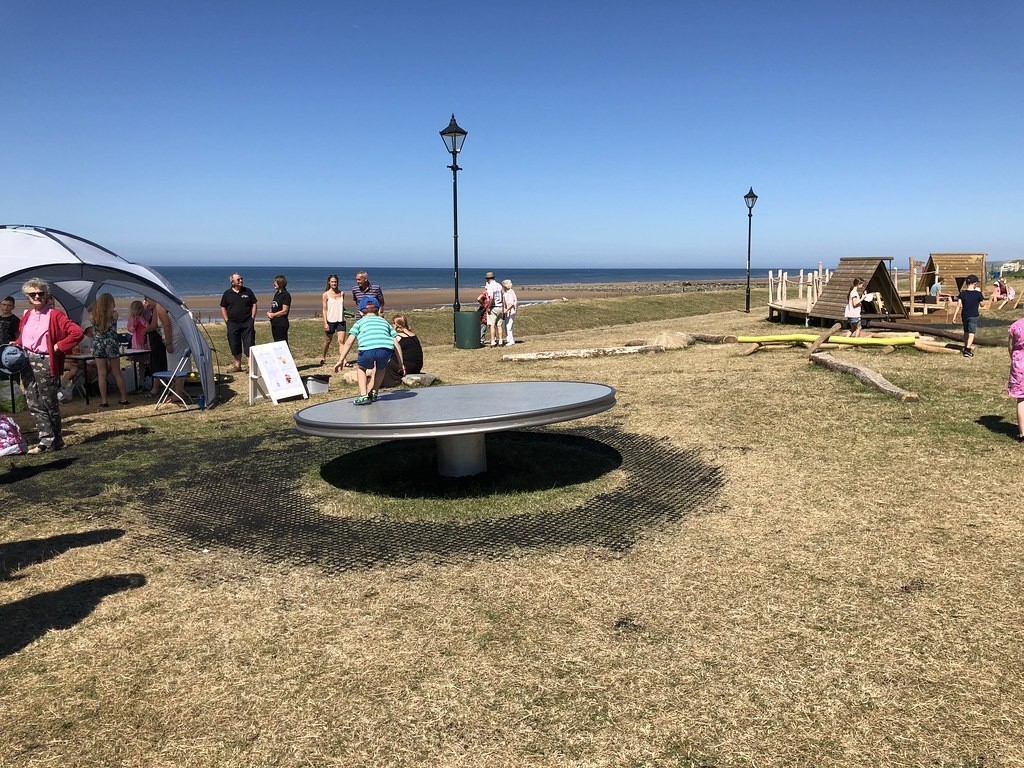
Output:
[152,346,195,411]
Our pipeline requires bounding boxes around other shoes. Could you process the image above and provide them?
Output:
[27,445,53,454]
[119,401,129,405]
[499,344,503,347]
[506,343,512,346]
[367,393,377,402]
[321,360,326,365]
[227,366,242,373]
[100,403,108,407]
[353,396,371,405]
[490,342,497,347]
[343,363,353,366]
[144,392,157,398]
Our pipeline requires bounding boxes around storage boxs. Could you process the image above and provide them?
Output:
[300,374,331,394]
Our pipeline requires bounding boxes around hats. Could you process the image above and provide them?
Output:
[359,297,379,310]
[485,272,494,279]
[0,344,27,374]
[82,320,94,332]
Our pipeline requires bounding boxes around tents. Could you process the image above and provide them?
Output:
[0,223,217,408]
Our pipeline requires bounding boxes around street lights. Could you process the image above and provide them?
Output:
[438,113,468,347]
[743,185,759,313]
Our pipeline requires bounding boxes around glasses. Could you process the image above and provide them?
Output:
[330,274,337,277]
[233,278,243,281]
[27,292,46,297]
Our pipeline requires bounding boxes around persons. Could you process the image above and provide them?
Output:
[128,301,152,393]
[994,279,1015,302]
[65,357,78,381]
[82,320,113,379]
[320,275,352,366]
[220,274,258,373]
[266,276,292,351]
[477,282,488,346]
[367,314,423,391]
[952,274,985,357]
[145,296,192,402]
[486,271,505,347]
[844,277,867,350]
[1008,318,1024,441]
[144,300,168,395]
[334,297,406,405]
[352,271,384,320]
[89,293,131,407]
[10,278,85,455]
[0,297,21,343]
[931,278,949,301]
[502,280,518,347]
[46,294,55,308]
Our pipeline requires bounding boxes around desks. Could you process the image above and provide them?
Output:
[65,348,152,405]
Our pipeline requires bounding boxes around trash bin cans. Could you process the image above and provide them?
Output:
[453,311,480,350]
